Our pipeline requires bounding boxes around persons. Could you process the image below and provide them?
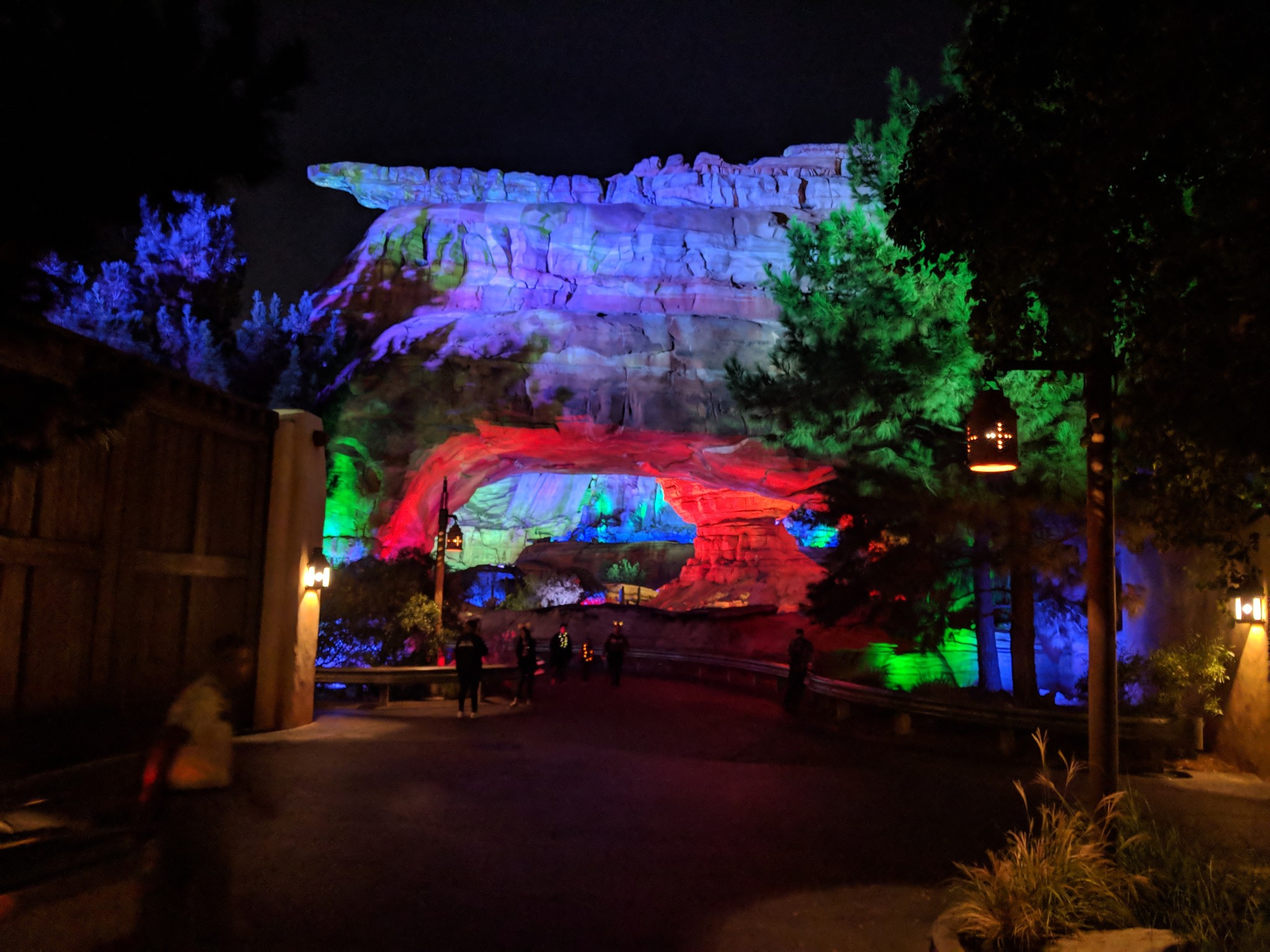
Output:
[506,626,537,708]
[452,618,489,718]
[602,624,632,688]
[130,633,255,952]
[574,636,597,682]
[548,624,571,684]
[783,627,815,713]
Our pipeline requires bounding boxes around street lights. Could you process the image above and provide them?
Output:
[966,336,1118,871]
[432,478,461,646]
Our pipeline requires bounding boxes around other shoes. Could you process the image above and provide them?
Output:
[510,700,518,707]
[525,700,533,706]
[458,711,465,719]
[470,712,480,719]
